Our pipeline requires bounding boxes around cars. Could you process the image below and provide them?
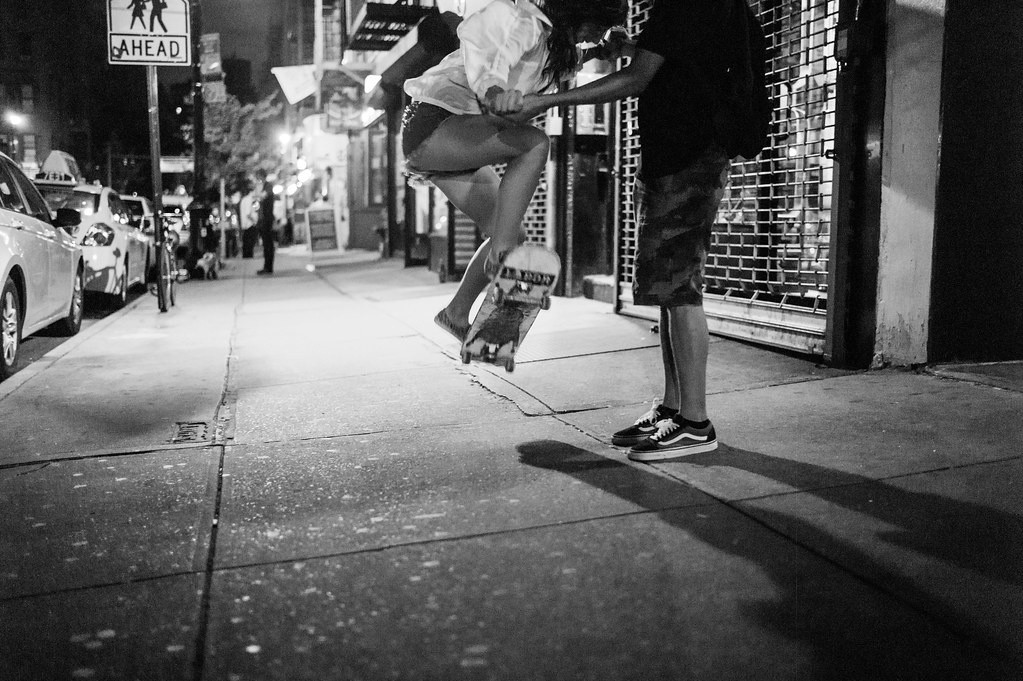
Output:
[26,149,153,311]
[0,151,91,370]
[119,156,239,264]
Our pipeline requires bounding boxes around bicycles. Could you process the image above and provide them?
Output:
[149,218,191,313]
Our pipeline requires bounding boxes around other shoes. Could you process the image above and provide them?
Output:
[433,309,471,343]
[483,256,500,281]
[257,270,272,275]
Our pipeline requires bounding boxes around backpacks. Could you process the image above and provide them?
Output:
[713,0,774,159]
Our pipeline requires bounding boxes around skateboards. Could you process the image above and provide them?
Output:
[459,241,561,373]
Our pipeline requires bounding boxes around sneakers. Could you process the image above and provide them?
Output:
[628,414,719,460]
[612,398,680,445]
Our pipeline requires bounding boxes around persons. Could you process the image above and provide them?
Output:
[254,170,275,274]
[484,0,733,461]
[402,0,631,343]
[240,189,257,259]
[225,205,237,258]
